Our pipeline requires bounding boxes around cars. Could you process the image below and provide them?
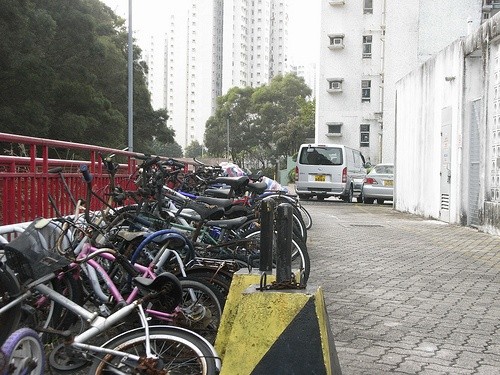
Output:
[363,163,394,205]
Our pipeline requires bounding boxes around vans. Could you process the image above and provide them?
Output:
[295,143,368,203]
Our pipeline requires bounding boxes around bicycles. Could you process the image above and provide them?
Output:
[0,149,313,375]
